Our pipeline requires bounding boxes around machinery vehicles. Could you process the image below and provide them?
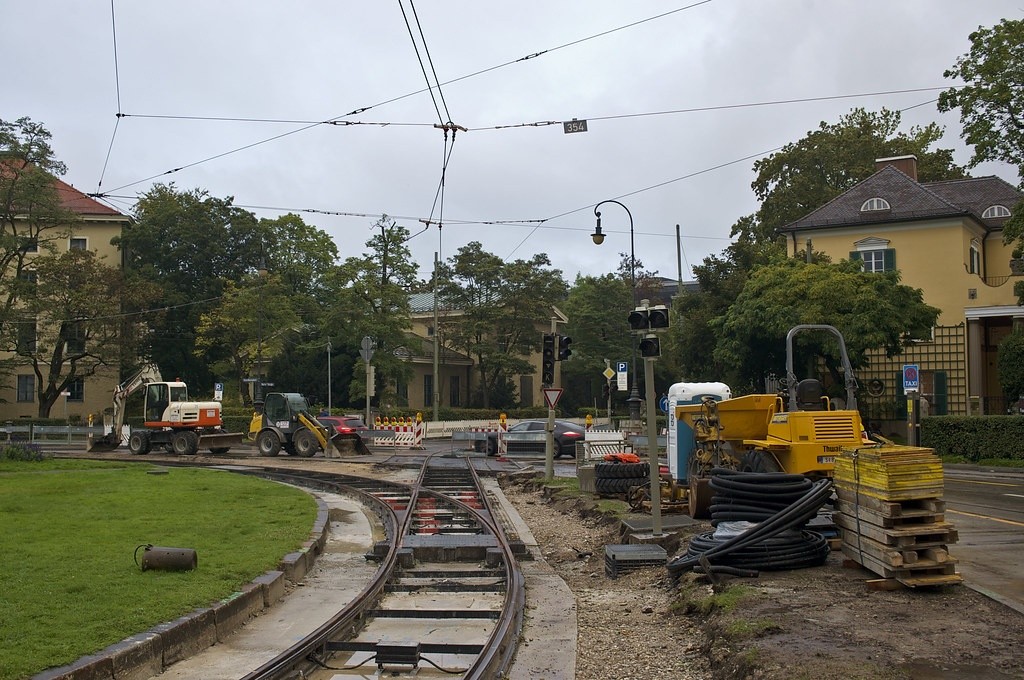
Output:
[675,324,879,493]
[248,392,373,458]
[87,363,245,455]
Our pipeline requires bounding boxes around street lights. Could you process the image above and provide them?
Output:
[590,200,643,421]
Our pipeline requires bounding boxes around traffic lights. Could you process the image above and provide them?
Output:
[558,336,573,361]
[542,334,556,384]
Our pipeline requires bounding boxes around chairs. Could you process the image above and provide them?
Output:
[158,394,180,420]
[275,408,288,421]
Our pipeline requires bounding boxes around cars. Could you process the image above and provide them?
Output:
[474,421,585,461]
[317,417,370,433]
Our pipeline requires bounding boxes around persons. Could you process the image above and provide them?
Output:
[319,408,329,417]
